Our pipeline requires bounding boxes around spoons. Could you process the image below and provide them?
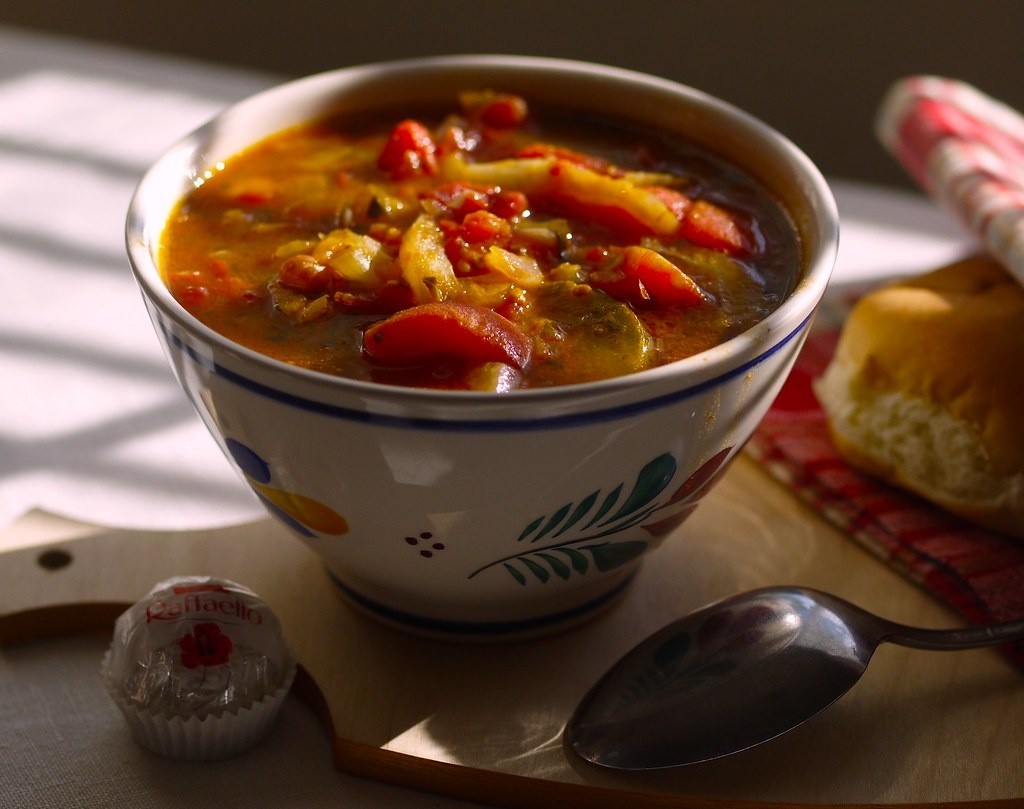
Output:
[562,586,1023,776]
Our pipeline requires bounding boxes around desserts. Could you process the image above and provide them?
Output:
[103,574,296,760]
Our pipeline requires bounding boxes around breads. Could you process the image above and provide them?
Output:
[810,251,1024,531]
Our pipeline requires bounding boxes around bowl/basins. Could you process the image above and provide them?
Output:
[124,55,842,644]
